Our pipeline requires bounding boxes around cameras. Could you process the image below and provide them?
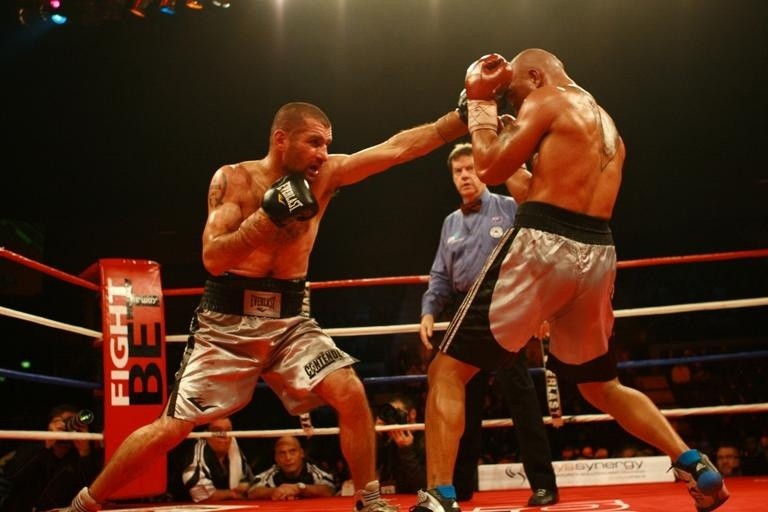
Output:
[62,409,94,433]
[378,404,406,425]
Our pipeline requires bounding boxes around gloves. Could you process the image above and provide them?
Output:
[455,52,513,125]
[261,174,318,228]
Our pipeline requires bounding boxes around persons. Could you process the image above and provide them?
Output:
[409,48,729,512]
[55,89,470,512]
[3,337,768,501]
[418,143,560,506]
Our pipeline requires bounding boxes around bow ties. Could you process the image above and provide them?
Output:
[460,199,481,214]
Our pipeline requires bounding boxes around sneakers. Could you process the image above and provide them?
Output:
[528,488,559,506]
[353,480,401,512]
[665,448,730,512]
[408,484,461,512]
